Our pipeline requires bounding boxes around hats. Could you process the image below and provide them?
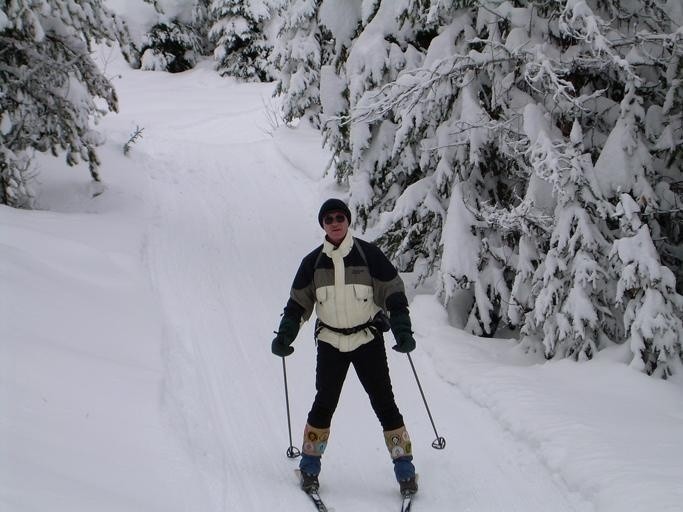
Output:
[318,198,351,230]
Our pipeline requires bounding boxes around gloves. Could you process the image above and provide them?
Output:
[271,337,294,356]
[392,331,416,353]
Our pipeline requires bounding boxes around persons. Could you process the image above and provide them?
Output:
[270,197,419,496]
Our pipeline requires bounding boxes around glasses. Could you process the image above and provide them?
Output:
[325,214,345,225]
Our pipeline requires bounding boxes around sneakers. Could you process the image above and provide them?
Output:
[395,463,418,495]
[301,454,321,491]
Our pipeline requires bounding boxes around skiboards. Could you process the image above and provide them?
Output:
[294,468,419,511]
